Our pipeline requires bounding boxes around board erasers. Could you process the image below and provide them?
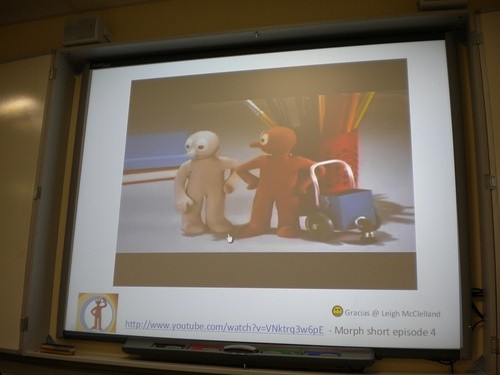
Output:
[224,344,260,352]
[39,343,80,357]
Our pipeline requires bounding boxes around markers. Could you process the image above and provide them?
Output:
[301,351,341,358]
[151,343,189,350]
[190,345,223,352]
[262,348,301,355]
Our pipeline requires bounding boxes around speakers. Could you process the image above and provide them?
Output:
[60,14,113,46]
